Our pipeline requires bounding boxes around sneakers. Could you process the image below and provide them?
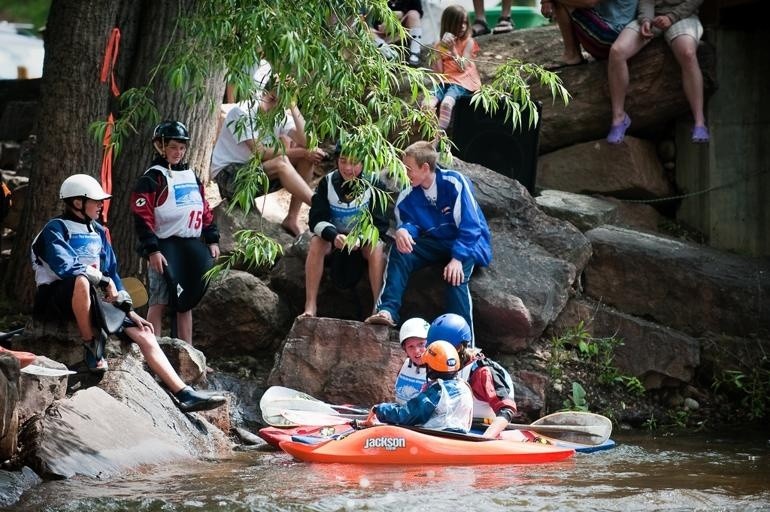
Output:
[175,385,226,412]
[409,52,423,66]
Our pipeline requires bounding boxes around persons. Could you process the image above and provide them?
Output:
[130,120,221,374]
[366,0,424,69]
[209,75,325,240]
[540,0,639,71]
[225,32,274,103]
[421,4,482,152]
[369,140,494,349]
[31,174,227,413]
[394,316,431,406]
[471,0,514,38]
[301,138,390,322]
[366,340,474,433]
[606,0,710,146]
[426,313,517,439]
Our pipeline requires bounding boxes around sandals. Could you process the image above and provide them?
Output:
[493,16,515,33]
[471,19,491,38]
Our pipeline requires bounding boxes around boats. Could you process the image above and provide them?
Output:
[279,425,575,465]
[258,403,615,454]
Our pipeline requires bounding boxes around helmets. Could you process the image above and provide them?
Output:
[399,318,430,345]
[59,174,113,201]
[427,313,471,346]
[153,121,191,140]
[420,340,460,372]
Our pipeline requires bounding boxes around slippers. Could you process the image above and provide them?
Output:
[692,126,709,143]
[540,57,588,70]
[606,113,632,144]
[364,310,396,327]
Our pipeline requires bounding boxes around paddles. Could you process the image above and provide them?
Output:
[260,385,373,428]
[19,363,78,376]
[472,411,612,445]
[0,278,147,338]
